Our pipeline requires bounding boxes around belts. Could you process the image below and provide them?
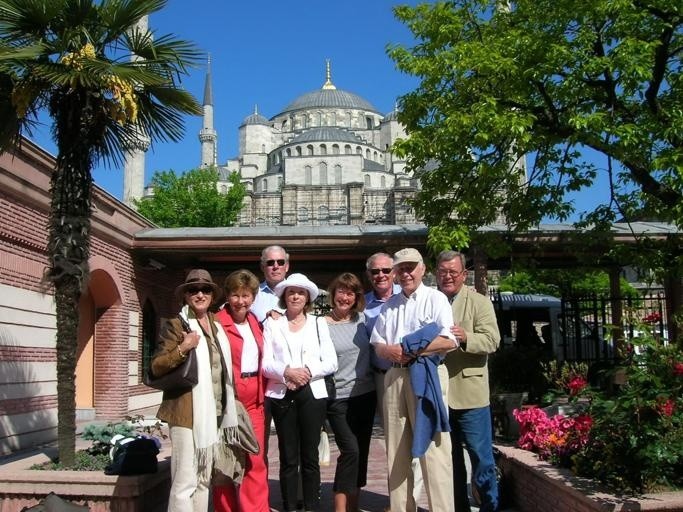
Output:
[389,362,407,368]
[374,368,388,374]
[241,371,261,377]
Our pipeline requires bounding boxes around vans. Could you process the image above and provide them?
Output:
[488,293,626,395]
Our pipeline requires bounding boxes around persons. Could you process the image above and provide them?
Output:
[214,266,271,511]
[362,251,403,419]
[366,247,457,511]
[434,250,501,511]
[259,271,340,509]
[219,244,300,511]
[264,270,378,511]
[147,266,233,511]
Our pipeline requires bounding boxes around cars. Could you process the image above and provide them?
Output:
[562,313,669,376]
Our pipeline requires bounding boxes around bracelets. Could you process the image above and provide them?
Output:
[177,344,188,360]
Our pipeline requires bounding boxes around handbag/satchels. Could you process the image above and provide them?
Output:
[104,435,159,476]
[144,349,198,391]
[323,374,337,404]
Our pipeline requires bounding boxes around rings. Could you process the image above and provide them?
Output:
[293,384,297,389]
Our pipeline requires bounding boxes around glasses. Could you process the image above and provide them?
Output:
[370,268,391,274]
[270,381,296,410]
[435,269,463,277]
[186,286,212,295]
[266,259,285,266]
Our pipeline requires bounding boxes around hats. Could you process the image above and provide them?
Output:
[391,248,423,268]
[275,273,319,302]
[175,270,222,304]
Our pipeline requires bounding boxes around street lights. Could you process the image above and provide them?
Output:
[311,286,330,317]
